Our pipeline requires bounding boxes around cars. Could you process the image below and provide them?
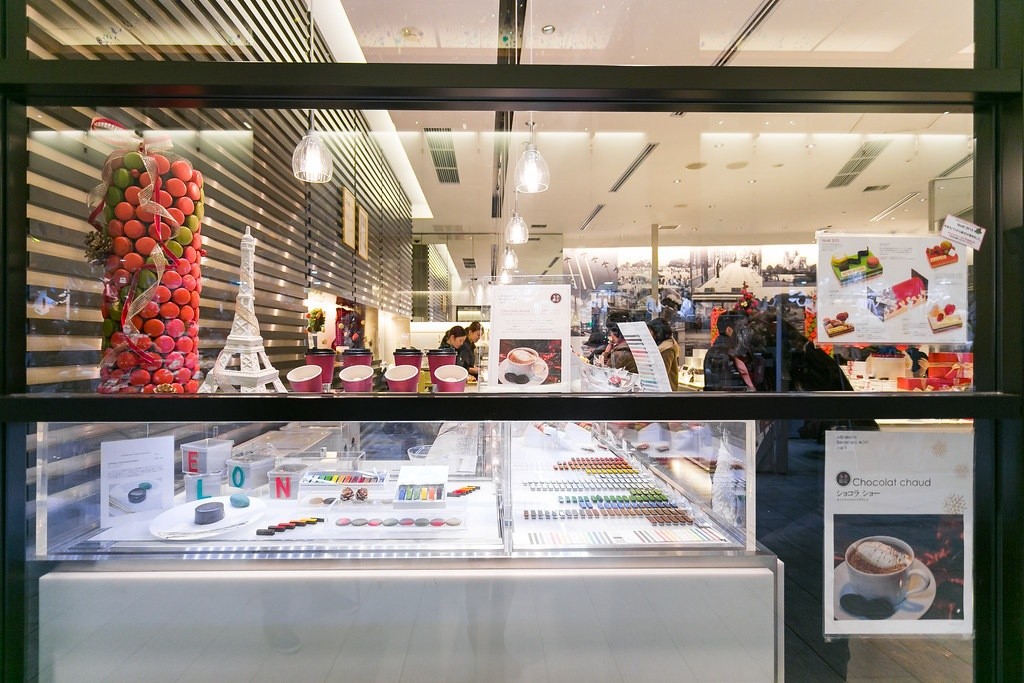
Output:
[741,261,748,267]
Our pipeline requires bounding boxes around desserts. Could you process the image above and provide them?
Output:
[139,483,152,490]
[229,493,250,507]
[127,488,146,503]
[194,501,224,525]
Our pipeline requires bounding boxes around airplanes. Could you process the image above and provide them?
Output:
[613,267,620,273]
[564,256,571,263]
[592,257,598,263]
[581,252,588,257]
[601,261,609,268]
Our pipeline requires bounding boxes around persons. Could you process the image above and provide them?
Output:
[833,344,929,380]
[584,311,681,391]
[438,320,484,381]
[704,310,878,682]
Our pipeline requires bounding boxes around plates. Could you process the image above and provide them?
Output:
[499,357,549,385]
[834,558,936,620]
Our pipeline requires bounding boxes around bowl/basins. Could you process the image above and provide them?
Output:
[407,445,455,466]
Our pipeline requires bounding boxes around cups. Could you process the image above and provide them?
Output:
[342,347,373,368]
[507,347,546,378]
[385,365,418,392]
[845,536,930,605]
[426,346,458,384]
[339,365,374,392]
[393,348,423,373]
[287,365,322,392]
[434,365,468,392]
[305,347,337,384]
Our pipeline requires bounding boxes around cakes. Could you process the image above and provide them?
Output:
[928,304,963,334]
[831,245,883,287]
[866,268,929,323]
[925,240,959,268]
[823,312,855,337]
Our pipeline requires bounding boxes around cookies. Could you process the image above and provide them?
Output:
[505,372,529,384]
[840,593,895,619]
